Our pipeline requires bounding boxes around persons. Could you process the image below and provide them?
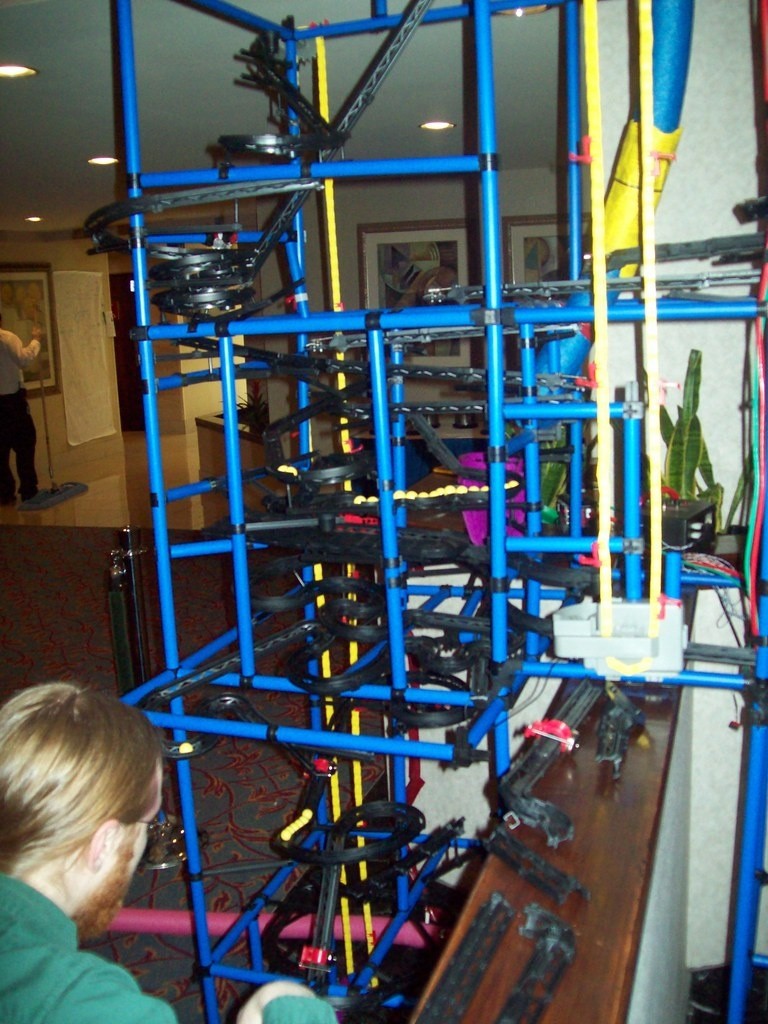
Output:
[0,314,42,504]
[0,683,338,1024]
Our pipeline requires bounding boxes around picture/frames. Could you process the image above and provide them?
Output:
[0,262,62,400]
[356,219,484,371]
[501,212,593,371]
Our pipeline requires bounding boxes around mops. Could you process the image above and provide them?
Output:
[17,305,88,511]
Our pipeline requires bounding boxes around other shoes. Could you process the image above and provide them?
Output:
[21,488,39,501]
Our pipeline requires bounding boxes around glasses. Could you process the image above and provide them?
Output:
[137,818,165,850]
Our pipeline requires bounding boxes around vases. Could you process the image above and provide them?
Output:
[195,405,266,491]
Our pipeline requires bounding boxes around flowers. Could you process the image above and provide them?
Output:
[237,378,268,433]
